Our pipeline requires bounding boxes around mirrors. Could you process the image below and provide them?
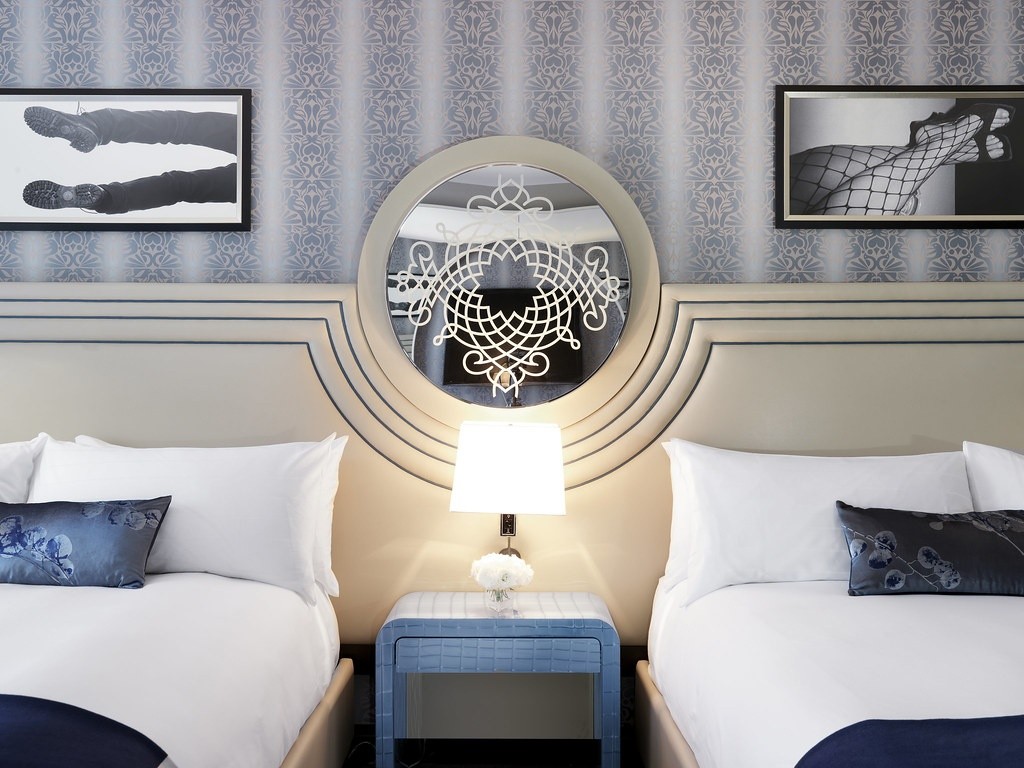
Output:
[356,134,661,427]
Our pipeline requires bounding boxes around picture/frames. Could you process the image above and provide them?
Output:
[386,272,436,318]
[600,278,629,314]
[0,88,252,232]
[774,85,1024,229]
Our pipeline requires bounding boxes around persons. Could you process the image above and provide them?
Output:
[790,102,1015,216]
[23,105,237,215]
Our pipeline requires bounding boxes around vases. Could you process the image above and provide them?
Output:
[486,591,514,617]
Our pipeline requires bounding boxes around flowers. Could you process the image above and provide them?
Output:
[470,553,534,601]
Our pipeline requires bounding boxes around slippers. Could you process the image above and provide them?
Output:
[910,102,1016,136]
[910,132,1013,166]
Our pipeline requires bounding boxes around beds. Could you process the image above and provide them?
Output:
[0,570,358,768]
[632,572,1024,768]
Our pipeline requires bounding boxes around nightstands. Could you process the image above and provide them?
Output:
[373,591,621,768]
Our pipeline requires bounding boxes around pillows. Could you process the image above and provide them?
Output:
[0,494,173,590]
[961,441,1024,512]
[26,432,337,607]
[672,434,977,607]
[835,500,1024,597]
[658,441,691,589]
[75,433,350,598]
[0,435,48,503]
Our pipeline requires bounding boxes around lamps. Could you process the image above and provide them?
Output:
[449,420,568,591]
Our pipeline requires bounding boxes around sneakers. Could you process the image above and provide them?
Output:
[23,106,100,154]
[22,181,113,214]
